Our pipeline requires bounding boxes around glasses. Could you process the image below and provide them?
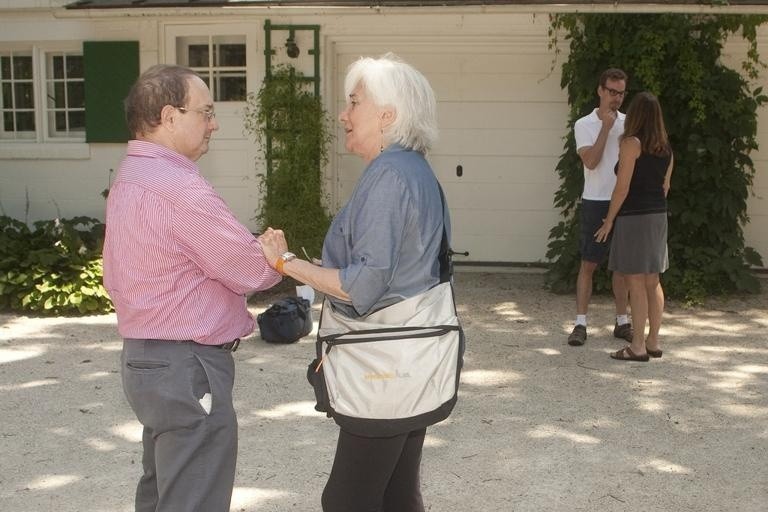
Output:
[602,84,628,98]
[174,103,219,122]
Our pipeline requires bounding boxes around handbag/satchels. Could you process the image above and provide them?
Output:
[308,274,469,441]
[258,296,315,345]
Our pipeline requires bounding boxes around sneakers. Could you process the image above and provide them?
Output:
[567,323,588,347]
[613,320,638,343]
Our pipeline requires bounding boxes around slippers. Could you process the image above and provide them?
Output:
[608,338,664,361]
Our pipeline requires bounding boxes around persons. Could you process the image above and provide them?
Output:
[592,90,675,362]
[256,50,453,512]
[567,68,635,346]
[99,64,290,511]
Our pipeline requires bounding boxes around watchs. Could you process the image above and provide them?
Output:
[274,251,296,276]
